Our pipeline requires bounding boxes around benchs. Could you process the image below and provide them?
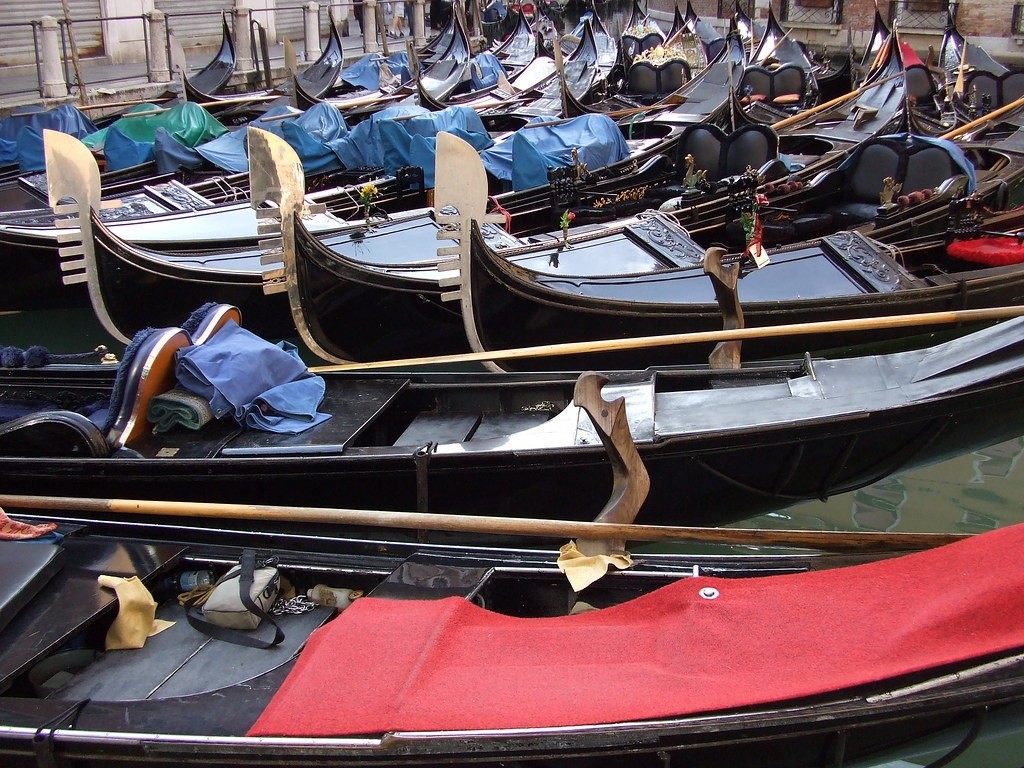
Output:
[0,544,184,684]
[220,381,413,458]
[255,563,496,737]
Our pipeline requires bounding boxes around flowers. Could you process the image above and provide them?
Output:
[358,184,379,214]
[559,210,578,231]
[741,192,769,241]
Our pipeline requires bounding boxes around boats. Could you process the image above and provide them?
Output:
[0,308,1024,510]
[431,66,1024,354]
[1,493,1024,768]
[0,0,1009,363]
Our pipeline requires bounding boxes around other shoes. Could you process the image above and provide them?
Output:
[360,33,363,36]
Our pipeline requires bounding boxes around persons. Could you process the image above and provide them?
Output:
[429,0,442,37]
[353,0,363,37]
[378,0,414,38]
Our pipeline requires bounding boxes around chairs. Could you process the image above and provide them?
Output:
[825,142,955,220]
[962,69,1024,120]
[739,62,806,107]
[659,124,779,195]
[701,37,726,65]
[618,33,664,64]
[624,58,692,101]
[904,65,937,111]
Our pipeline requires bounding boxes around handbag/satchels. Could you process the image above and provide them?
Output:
[184,549,285,648]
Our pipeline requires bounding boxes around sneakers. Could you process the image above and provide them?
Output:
[389,30,396,38]
[400,31,405,37]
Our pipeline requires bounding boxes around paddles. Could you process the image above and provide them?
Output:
[725,40,737,130]
[759,27,795,66]
[374,96,534,123]
[769,70,906,129]
[952,35,968,94]
[749,17,755,62]
[665,18,693,47]
[938,98,1024,140]
[524,103,678,130]
[306,306,1024,375]
[0,495,978,554]
[286,94,407,119]
